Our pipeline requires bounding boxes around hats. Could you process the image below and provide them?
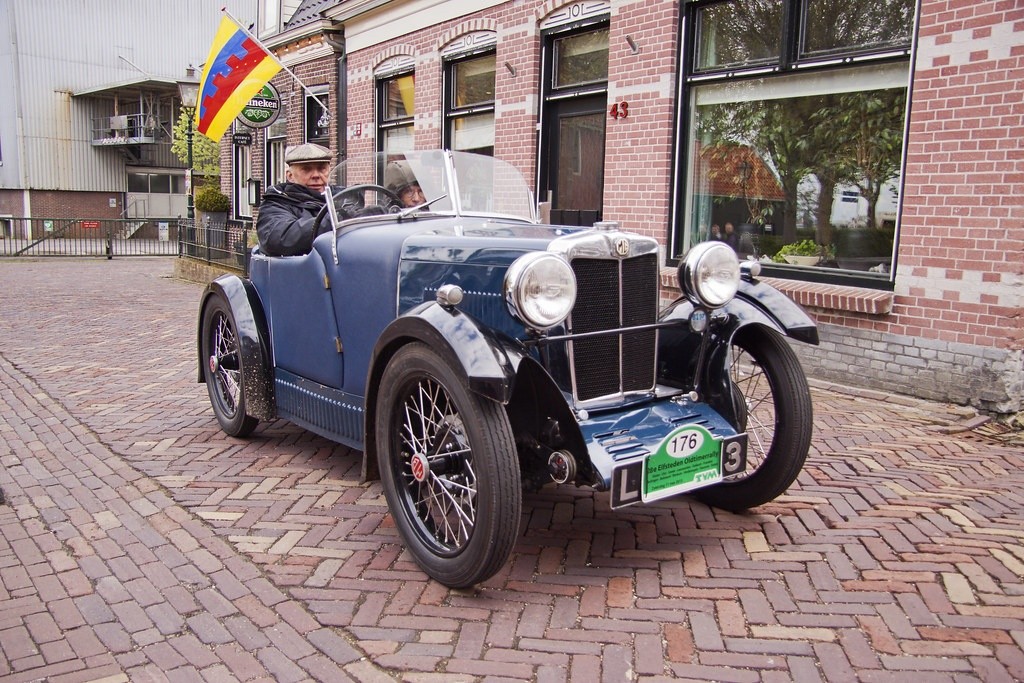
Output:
[286,143,333,164]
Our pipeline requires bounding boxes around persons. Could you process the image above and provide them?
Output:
[384,160,435,213]
[712,223,738,251]
[255,143,365,256]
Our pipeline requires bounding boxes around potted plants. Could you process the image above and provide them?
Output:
[772,240,836,266]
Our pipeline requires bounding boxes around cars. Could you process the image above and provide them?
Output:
[190,147,823,592]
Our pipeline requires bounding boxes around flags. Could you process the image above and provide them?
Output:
[398,75,464,133]
[194,14,284,144]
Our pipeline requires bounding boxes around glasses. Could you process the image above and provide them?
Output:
[400,186,425,199]
